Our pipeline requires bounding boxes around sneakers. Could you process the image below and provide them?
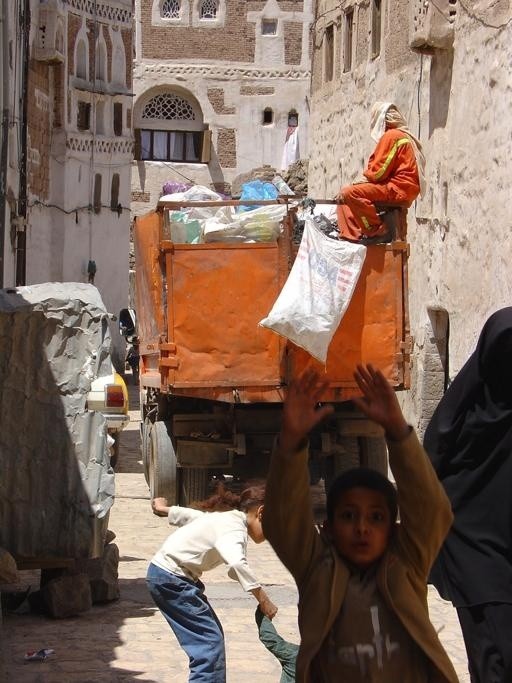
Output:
[359,234,393,244]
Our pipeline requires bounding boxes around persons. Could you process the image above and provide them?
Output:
[420,307,512,683]
[146,483,278,683]
[125,337,139,376]
[254,604,299,683]
[334,102,421,245]
[263,360,461,683]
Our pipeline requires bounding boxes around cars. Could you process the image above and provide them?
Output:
[86,363,129,468]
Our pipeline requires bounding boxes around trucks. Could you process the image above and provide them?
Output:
[119,199,415,508]
